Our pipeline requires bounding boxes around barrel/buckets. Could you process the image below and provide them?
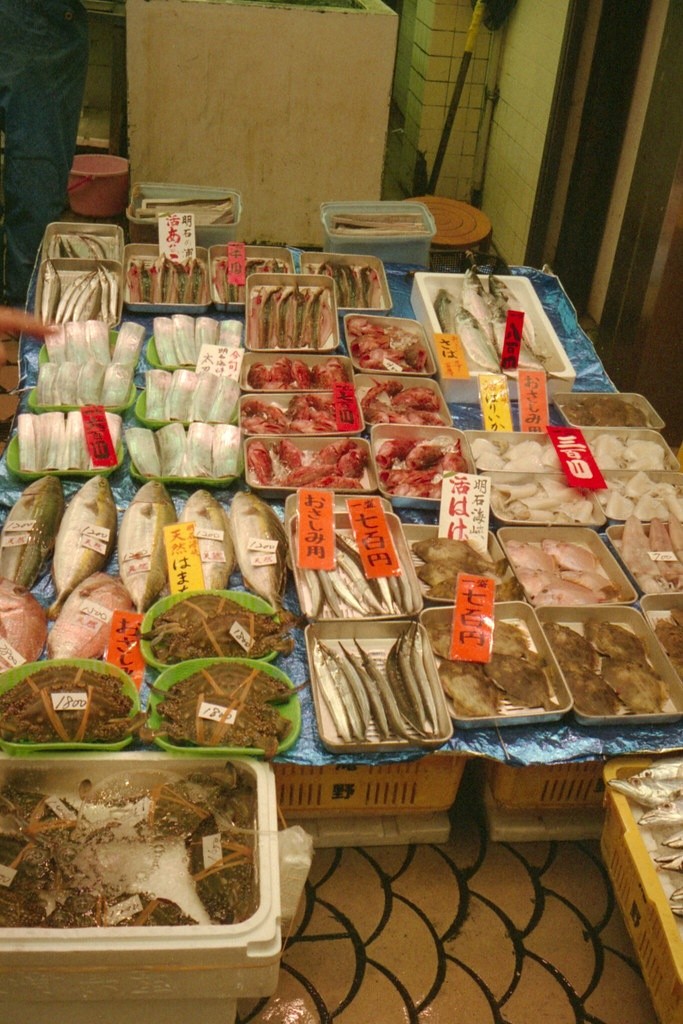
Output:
[320,201,438,269]
[66,153,130,216]
[127,182,244,246]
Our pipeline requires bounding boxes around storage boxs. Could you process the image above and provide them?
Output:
[245,272,342,355]
[418,601,575,730]
[605,522,683,594]
[352,373,454,435]
[299,251,393,320]
[238,351,356,393]
[238,392,366,437]
[121,243,212,314]
[463,429,565,473]
[409,270,578,405]
[639,592,683,683]
[479,471,608,533]
[305,620,453,757]
[495,525,639,606]
[125,181,244,249]
[319,200,437,269]
[369,421,479,512]
[33,257,123,330]
[401,522,526,607]
[581,429,681,471]
[41,222,125,265]
[534,604,683,728]
[0,748,683,1024]
[594,472,683,526]
[344,312,438,379]
[243,436,378,501]
[287,509,424,622]
[207,244,296,315]
[551,391,666,434]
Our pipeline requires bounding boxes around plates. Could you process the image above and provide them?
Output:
[6,434,125,480]
[28,382,136,415]
[146,334,196,372]
[39,330,139,370]
[130,458,244,489]
[134,389,240,429]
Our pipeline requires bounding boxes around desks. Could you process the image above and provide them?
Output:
[76,4,127,159]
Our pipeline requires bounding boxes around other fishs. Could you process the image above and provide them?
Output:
[231,491,294,623]
[48,573,136,661]
[0,578,46,673]
[410,537,683,716]
[119,480,178,613]
[637,800,683,918]
[312,617,440,742]
[48,474,117,621]
[0,475,66,588]
[127,254,382,349]
[433,270,568,385]
[607,757,683,808]
[241,317,468,498]
[184,490,236,592]
[292,519,414,618]
[41,235,118,326]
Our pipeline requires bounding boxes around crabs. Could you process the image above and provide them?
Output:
[0,666,152,744]
[145,666,310,761]
[134,593,307,661]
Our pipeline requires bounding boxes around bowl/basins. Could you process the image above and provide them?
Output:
[139,590,280,673]
[0,658,140,752]
[146,657,300,755]
